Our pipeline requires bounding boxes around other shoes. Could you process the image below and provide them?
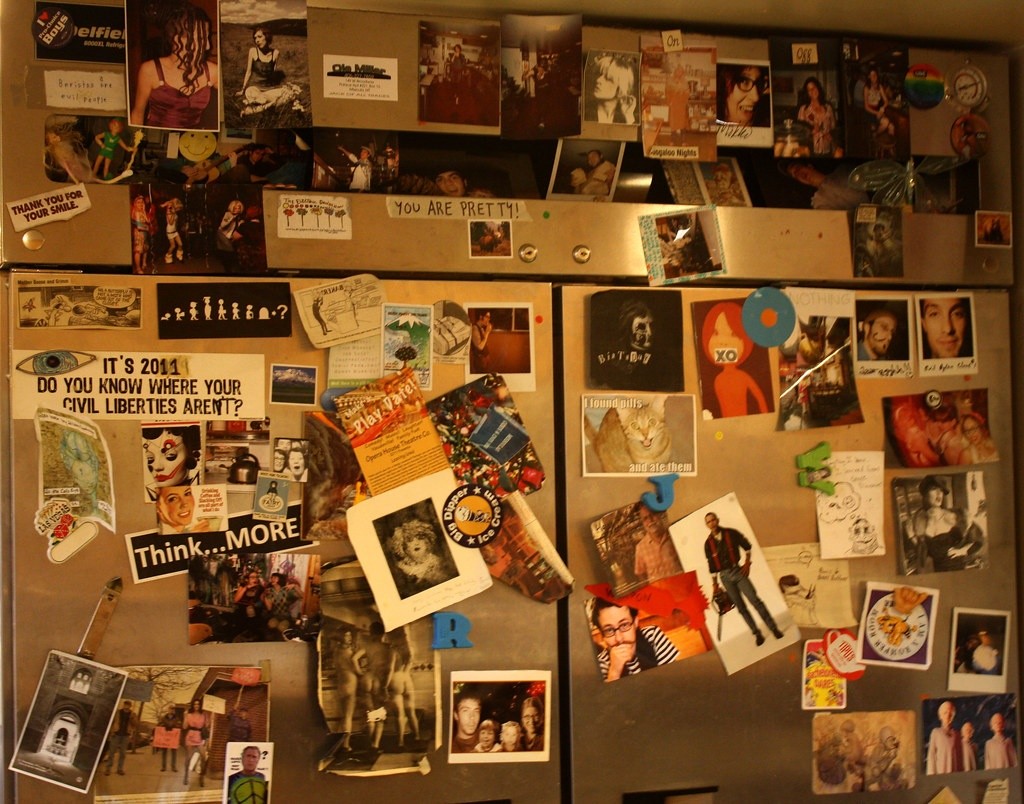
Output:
[756,636,764,646]
[117,770,125,775]
[172,768,178,771]
[161,768,166,772]
[773,630,783,639]
[184,780,189,785]
[199,777,205,787]
[104,770,110,776]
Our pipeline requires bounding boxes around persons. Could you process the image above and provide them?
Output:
[333,623,421,750]
[863,69,894,136]
[633,503,681,582]
[451,44,465,64]
[105,698,266,804]
[473,310,492,371]
[140,425,308,632]
[932,404,995,464]
[335,144,467,197]
[450,693,545,753]
[176,137,282,183]
[235,26,303,117]
[665,62,692,148]
[800,76,844,158]
[131,5,218,129]
[778,159,870,209]
[702,512,783,647]
[588,56,637,124]
[717,64,768,124]
[577,149,616,195]
[904,475,984,575]
[132,197,243,274]
[857,311,896,361]
[921,297,966,359]
[93,120,132,178]
[591,595,681,683]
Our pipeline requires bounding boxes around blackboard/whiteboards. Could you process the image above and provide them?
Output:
[1,260,1024,804]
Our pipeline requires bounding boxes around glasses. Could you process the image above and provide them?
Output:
[247,575,261,580]
[962,421,983,436]
[602,620,635,639]
[736,77,770,94]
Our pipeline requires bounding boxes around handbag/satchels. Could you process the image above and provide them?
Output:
[202,727,210,740]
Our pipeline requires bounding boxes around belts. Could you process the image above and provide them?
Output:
[190,727,202,731]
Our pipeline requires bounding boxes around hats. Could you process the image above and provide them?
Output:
[919,475,951,496]
[862,306,899,332]
